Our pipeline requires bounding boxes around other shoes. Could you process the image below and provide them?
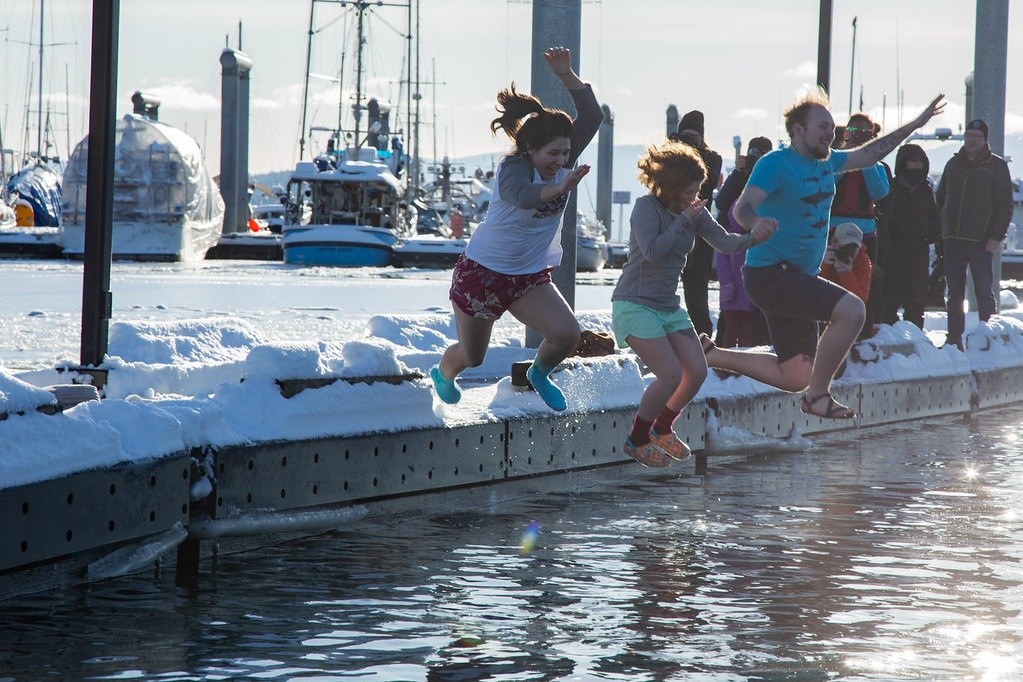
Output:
[649,427,690,460]
[937,336,964,352]
[623,436,671,469]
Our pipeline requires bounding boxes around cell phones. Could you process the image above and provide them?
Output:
[849,129,872,145]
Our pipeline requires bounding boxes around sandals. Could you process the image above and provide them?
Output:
[802,392,854,419]
[698,332,715,354]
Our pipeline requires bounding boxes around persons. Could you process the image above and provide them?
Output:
[698,94,948,419]
[715,137,771,348]
[611,141,779,468]
[935,119,1014,353]
[818,222,873,342]
[430,46,604,411]
[678,111,723,340]
[830,114,943,331]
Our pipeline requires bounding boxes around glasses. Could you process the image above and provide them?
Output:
[845,127,873,141]
[964,131,984,140]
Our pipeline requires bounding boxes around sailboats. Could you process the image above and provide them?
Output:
[0,0,83,252]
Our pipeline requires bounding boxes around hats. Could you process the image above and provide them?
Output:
[748,136,772,150]
[678,111,704,139]
[965,119,989,140]
[835,222,864,247]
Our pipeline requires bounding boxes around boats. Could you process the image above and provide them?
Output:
[248,0,613,278]
[897,128,1023,290]
[62,97,228,263]
[610,242,631,265]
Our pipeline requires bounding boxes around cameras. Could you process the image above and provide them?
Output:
[742,153,763,170]
[829,247,850,264]
